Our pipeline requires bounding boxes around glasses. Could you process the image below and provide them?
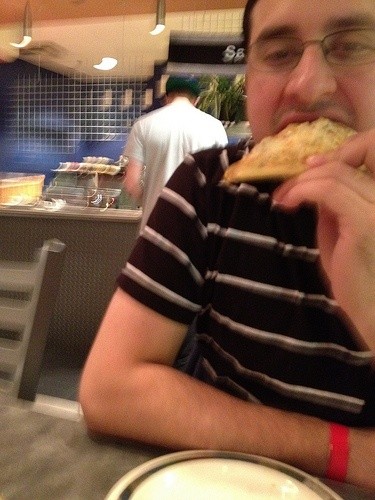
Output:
[244,28,374,72]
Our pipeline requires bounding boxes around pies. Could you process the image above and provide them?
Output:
[220,115,369,180]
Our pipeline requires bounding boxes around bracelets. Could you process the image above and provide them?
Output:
[324,421,350,482]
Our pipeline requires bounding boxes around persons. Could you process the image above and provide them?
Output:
[78,0,375,493]
[122,75,229,241]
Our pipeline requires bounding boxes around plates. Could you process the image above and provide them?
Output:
[103,450,345,500]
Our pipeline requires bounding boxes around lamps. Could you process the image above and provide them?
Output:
[10,1,32,48]
[149,0,166,36]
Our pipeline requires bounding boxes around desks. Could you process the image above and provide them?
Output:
[0,396,375,500]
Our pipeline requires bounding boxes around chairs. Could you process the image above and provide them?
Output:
[0,239,66,399]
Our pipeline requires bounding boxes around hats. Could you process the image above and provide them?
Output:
[166,76,200,97]
